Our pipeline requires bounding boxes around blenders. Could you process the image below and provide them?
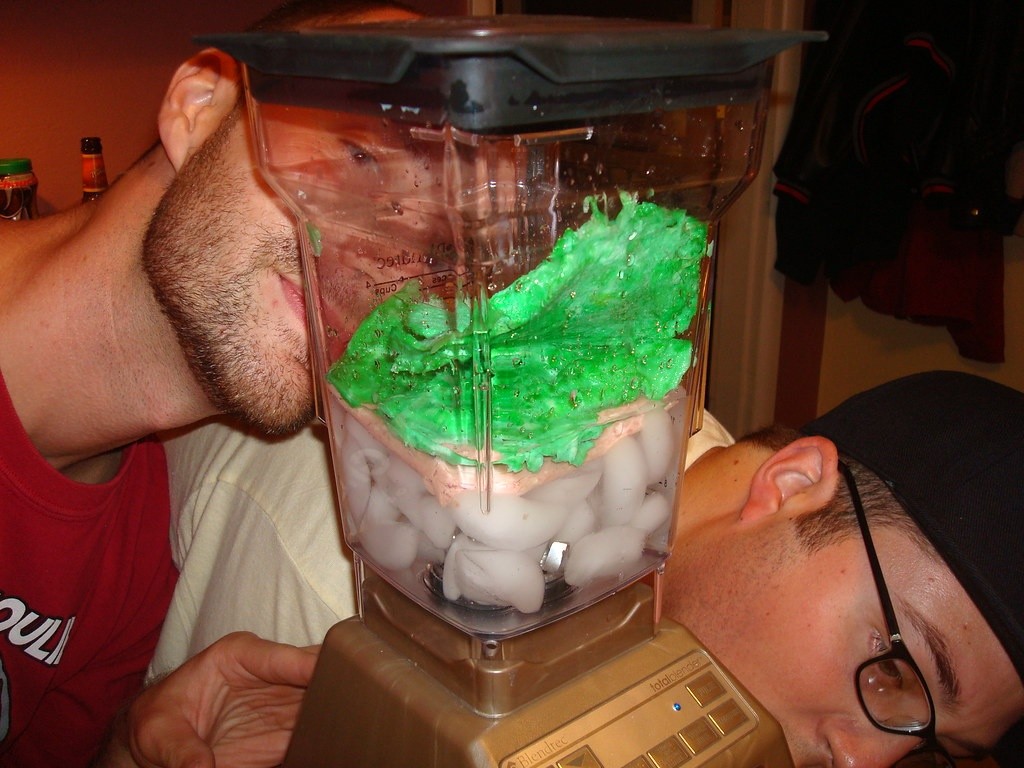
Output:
[184,25,830,768]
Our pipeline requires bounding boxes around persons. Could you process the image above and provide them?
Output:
[0,0,527,768]
[143,371,1024,768]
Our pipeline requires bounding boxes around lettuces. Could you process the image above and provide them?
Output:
[325,190,707,473]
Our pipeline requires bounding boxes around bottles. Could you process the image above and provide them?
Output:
[80,135,108,200]
[0,158,38,221]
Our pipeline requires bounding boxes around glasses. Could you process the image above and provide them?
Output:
[837,461,958,768]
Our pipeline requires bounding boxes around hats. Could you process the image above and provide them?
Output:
[801,370,1024,687]
